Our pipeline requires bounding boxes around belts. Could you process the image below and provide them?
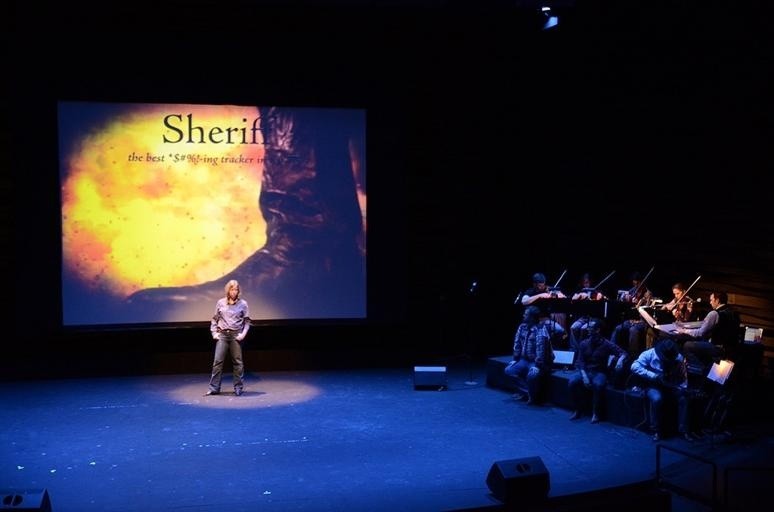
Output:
[218,329,241,335]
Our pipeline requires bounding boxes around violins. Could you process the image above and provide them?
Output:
[546,284,694,322]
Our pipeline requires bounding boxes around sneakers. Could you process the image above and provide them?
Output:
[527,394,534,405]
[234,388,242,396]
[590,414,598,423]
[205,389,220,396]
[569,411,581,420]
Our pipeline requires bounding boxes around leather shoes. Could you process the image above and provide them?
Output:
[684,433,694,441]
[653,432,660,441]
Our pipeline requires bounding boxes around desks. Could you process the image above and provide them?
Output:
[654,438,772,511]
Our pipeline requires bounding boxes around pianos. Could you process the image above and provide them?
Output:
[654,320,706,342]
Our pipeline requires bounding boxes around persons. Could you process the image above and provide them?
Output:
[201,279,251,398]
[521,274,566,346]
[630,338,688,441]
[660,284,695,324]
[567,319,629,423]
[571,275,604,351]
[611,275,653,352]
[506,305,554,404]
[677,289,736,365]
